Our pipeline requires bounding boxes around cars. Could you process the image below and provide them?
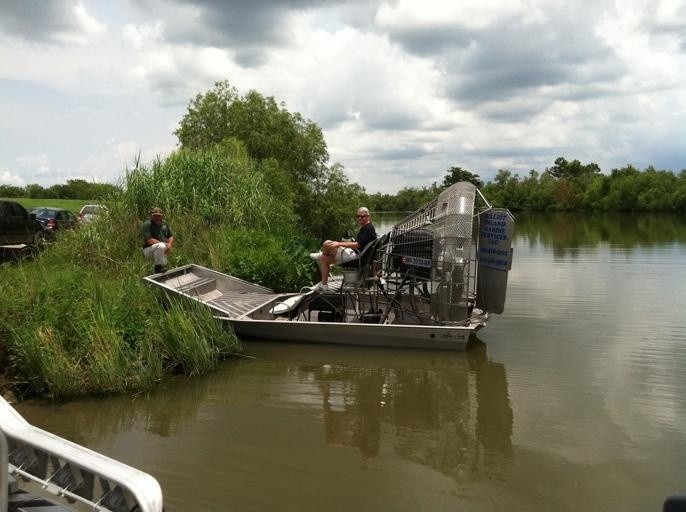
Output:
[28,206,83,236]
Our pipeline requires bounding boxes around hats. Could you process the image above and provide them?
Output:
[152,206,163,216]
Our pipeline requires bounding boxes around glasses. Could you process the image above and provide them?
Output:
[357,213,366,218]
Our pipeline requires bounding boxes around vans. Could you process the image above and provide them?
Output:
[0,200,46,259]
[77,204,113,230]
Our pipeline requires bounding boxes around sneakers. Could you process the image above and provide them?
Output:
[309,251,324,260]
[155,264,167,274]
[310,282,329,292]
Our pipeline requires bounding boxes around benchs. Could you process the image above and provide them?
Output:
[268,232,392,324]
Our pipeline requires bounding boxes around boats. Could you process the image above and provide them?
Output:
[0,394,163,512]
[215,334,514,492]
[141,180,516,351]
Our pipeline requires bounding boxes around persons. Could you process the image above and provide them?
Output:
[309,206,376,291]
[141,207,174,273]
